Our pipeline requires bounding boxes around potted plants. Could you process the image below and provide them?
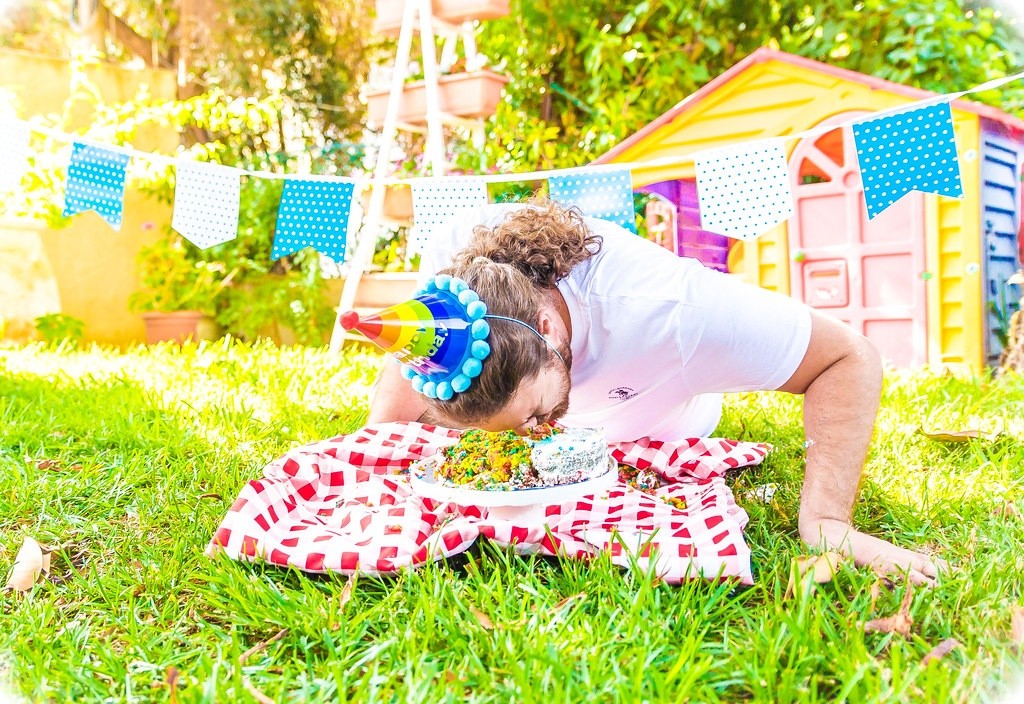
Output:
[128,235,227,344]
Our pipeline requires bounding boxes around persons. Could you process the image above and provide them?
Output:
[340,194,962,588]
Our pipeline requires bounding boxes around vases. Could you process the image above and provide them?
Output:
[364,71,511,131]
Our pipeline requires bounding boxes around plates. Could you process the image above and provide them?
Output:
[408,454,618,507]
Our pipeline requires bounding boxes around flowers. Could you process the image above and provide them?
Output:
[368,51,504,90]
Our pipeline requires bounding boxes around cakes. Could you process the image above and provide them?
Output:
[431,424,610,492]
